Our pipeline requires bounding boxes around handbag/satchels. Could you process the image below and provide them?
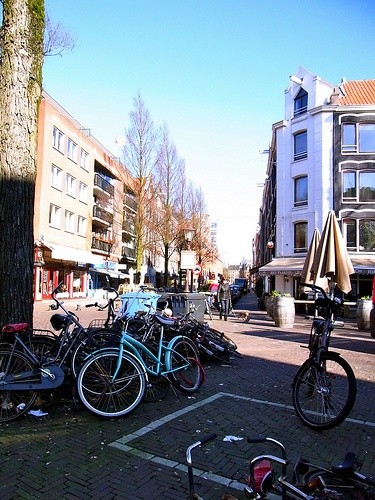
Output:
[228,301,233,314]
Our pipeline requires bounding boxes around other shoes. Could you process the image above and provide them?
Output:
[225,318,227,321]
[220,313,222,320]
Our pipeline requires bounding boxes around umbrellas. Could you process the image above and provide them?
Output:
[311,210,355,320]
[298,228,330,316]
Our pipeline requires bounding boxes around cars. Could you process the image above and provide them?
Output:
[230,284,240,295]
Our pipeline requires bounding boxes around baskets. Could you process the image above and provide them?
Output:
[87,319,110,330]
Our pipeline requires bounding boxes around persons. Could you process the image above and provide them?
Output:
[208,277,231,321]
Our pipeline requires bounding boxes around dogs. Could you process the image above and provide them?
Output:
[239,312,251,324]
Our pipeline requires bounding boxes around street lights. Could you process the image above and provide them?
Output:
[182,228,197,292]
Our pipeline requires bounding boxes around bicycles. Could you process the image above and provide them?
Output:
[293,281,358,433]
[185,432,375,500]
[0,280,244,423]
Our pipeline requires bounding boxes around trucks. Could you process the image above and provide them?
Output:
[235,278,248,294]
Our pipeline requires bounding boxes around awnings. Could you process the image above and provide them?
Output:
[41,241,103,265]
[259,255,375,277]
[97,269,129,278]
